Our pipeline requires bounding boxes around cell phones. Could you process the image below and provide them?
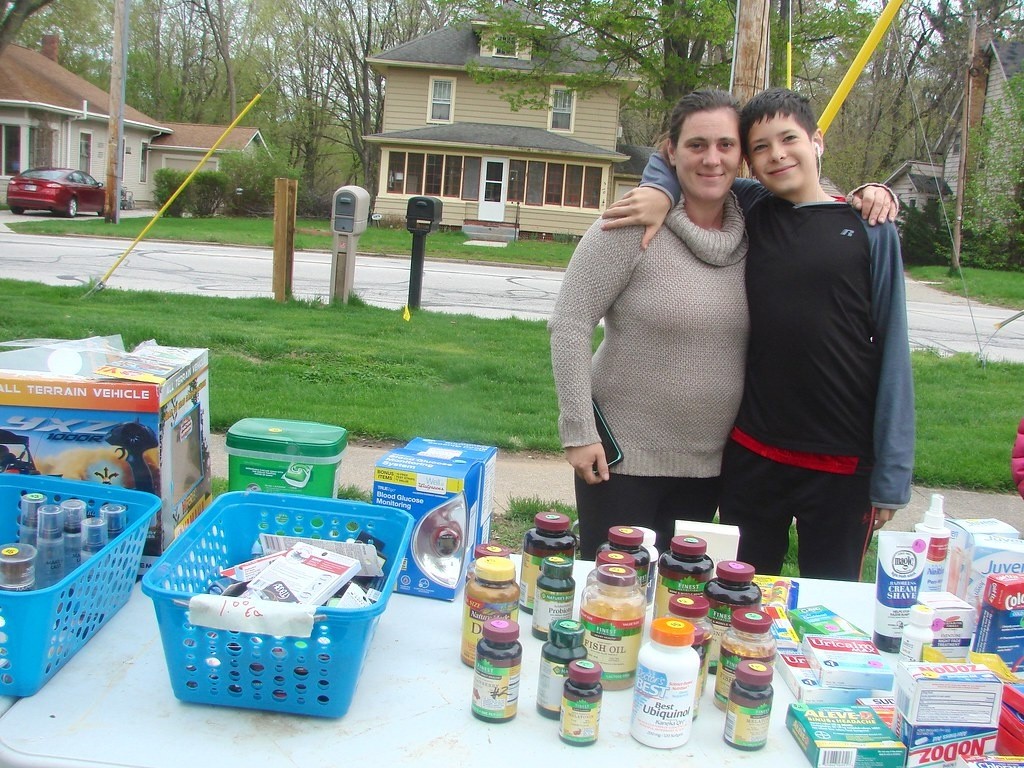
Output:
[591,398,625,475]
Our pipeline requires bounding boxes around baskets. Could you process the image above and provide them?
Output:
[142,490,414,719]
[0,473,163,696]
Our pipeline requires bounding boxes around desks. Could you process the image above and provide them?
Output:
[0,571,882,768]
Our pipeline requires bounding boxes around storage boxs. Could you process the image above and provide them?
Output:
[366,454,485,598]
[0,465,162,699]
[404,436,498,562]
[0,339,213,577]
[225,414,348,499]
[141,489,415,718]
[675,515,1024,768]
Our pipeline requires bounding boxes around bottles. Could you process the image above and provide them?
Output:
[0,493,127,591]
[899,604,935,662]
[724,660,773,751]
[587,551,641,589]
[630,617,700,748]
[519,512,577,615]
[532,555,575,641]
[703,561,763,675]
[537,620,587,720]
[912,493,950,593]
[652,535,714,619]
[596,526,651,597]
[663,594,714,722]
[714,609,776,711]
[471,618,522,723]
[580,564,647,691]
[630,527,659,610]
[558,659,603,746]
[461,544,520,669]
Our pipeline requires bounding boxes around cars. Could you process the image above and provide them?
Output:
[6,167,105,218]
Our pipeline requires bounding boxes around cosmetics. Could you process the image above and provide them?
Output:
[873,530,932,653]
[915,495,952,592]
[0,493,126,615]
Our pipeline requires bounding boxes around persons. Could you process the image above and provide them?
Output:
[547,86,899,564]
[601,88,915,582]
